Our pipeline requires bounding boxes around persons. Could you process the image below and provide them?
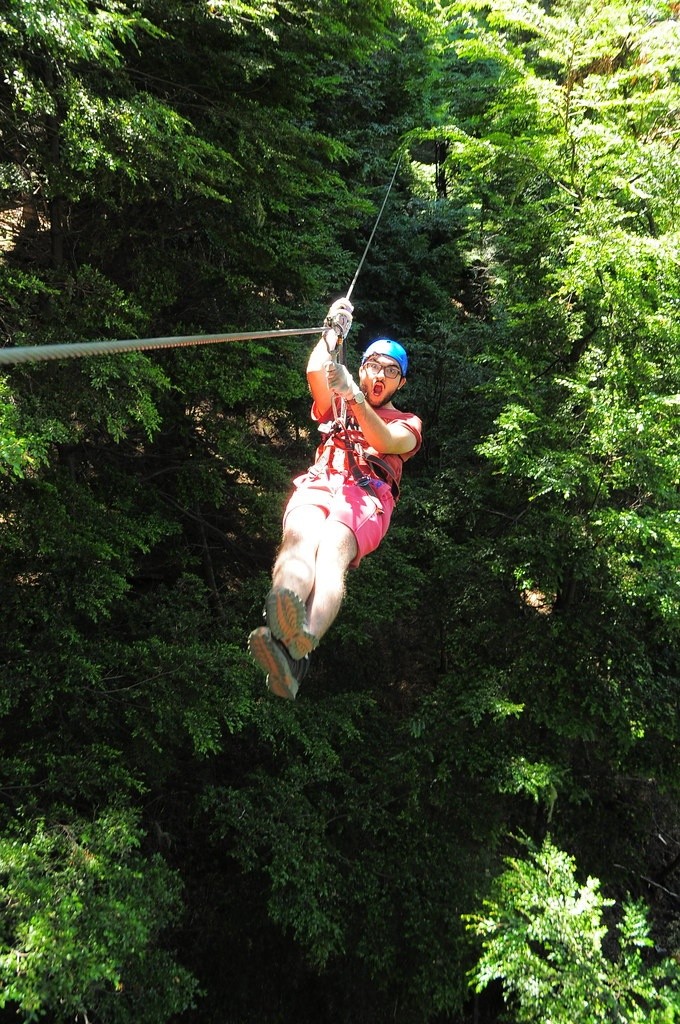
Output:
[248,296,420,699]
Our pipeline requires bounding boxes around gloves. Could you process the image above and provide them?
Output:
[324,297,354,340]
[323,361,360,402]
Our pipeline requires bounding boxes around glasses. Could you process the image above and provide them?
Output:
[362,361,402,379]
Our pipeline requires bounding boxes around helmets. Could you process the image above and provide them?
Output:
[360,339,409,375]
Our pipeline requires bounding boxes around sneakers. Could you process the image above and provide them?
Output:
[263,585,318,661]
[247,626,309,700]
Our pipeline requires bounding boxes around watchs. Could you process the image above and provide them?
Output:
[345,387,365,406]
